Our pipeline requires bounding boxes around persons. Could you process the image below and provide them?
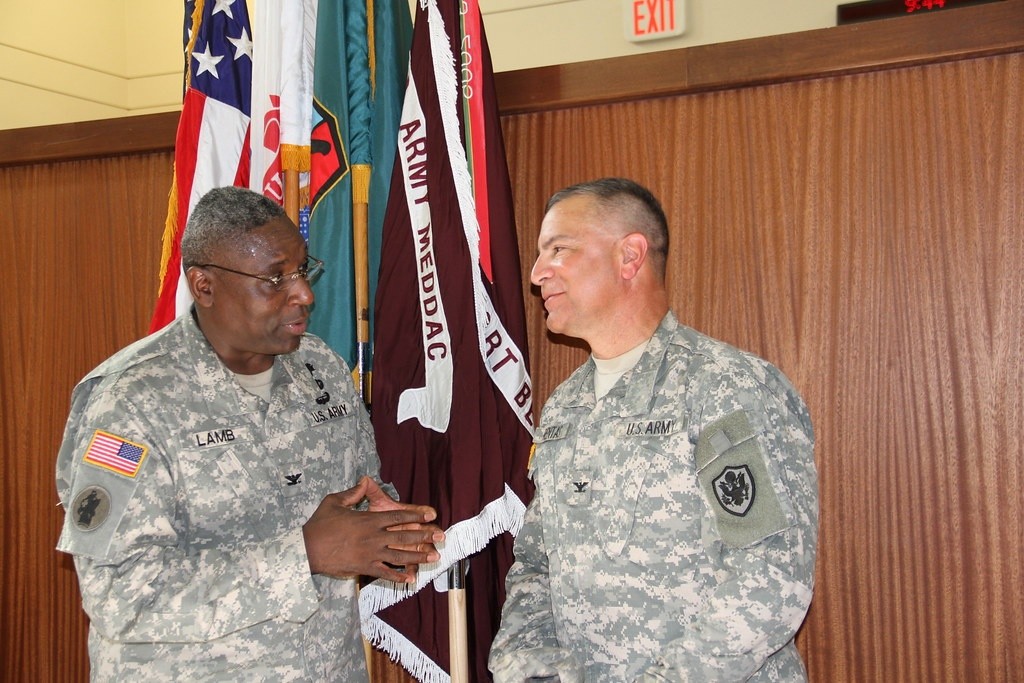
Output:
[54,187,445,683]
[488,176,820,683]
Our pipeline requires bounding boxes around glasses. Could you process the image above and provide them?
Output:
[195,254,324,292]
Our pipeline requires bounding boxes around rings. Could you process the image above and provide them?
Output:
[416,544,421,552]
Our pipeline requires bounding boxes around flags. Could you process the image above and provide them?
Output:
[149,0,415,394]
[351,0,536,683]
